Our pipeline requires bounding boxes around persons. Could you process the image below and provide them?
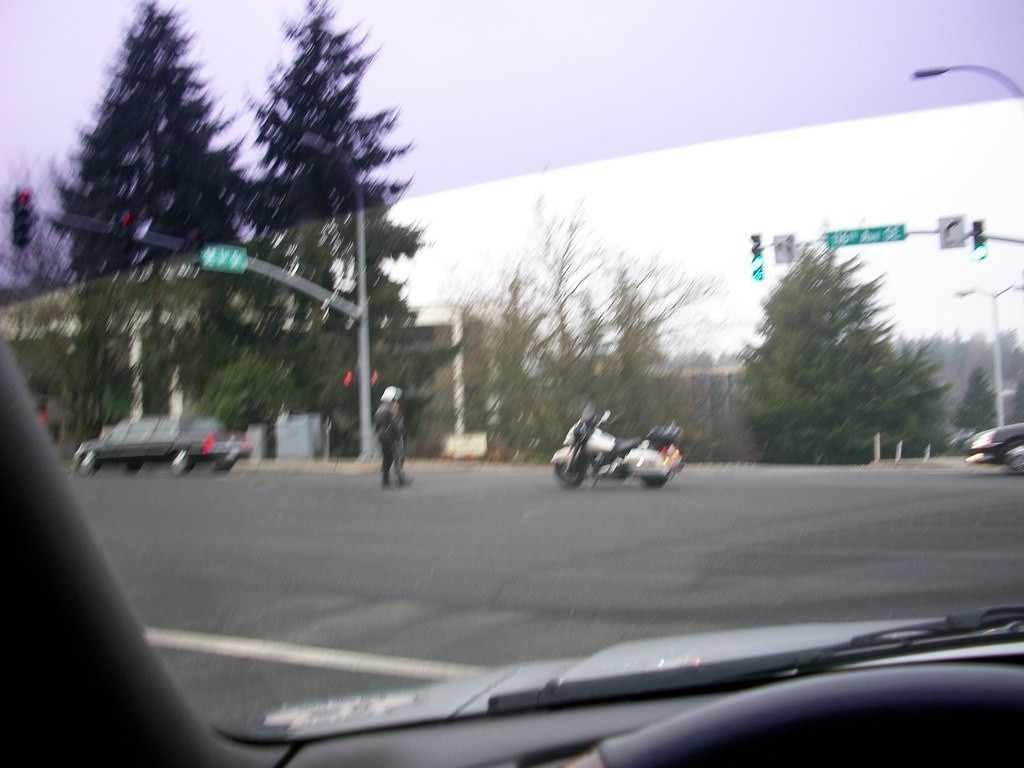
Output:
[371,385,416,488]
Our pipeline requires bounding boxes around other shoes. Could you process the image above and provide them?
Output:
[398,479,412,486]
[381,478,393,486]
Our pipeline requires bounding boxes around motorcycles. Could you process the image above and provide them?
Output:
[550,400,685,491]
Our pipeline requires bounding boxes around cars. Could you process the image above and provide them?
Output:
[962,422,1024,477]
[72,417,248,477]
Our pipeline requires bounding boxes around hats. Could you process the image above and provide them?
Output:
[380,387,397,403]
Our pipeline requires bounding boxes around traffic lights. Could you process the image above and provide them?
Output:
[750,234,765,283]
[11,188,35,246]
[970,219,987,260]
